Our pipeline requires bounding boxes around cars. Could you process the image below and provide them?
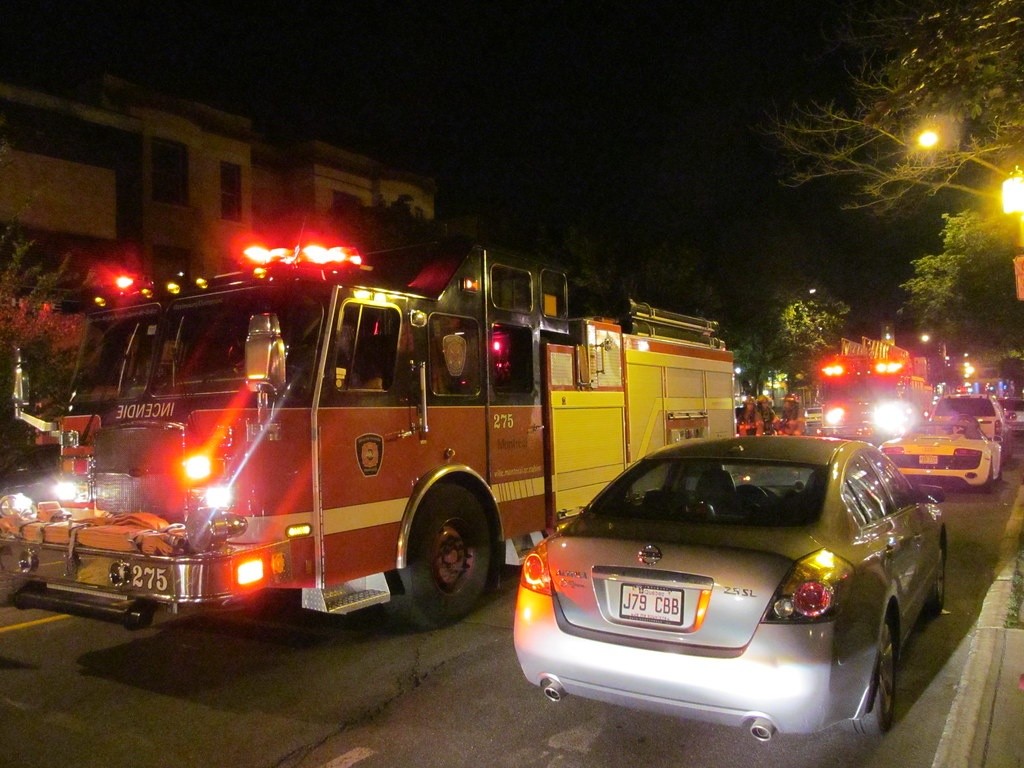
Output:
[512,434,952,735]
[804,406,823,426]
[1001,397,1024,437]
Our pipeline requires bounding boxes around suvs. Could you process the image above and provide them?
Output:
[933,394,1016,464]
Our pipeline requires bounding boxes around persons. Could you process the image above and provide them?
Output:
[772,392,808,436]
[755,394,778,437]
[737,395,765,437]
[335,320,386,390]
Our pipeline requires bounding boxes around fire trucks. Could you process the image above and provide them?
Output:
[817,337,937,447]
[0,254,738,635]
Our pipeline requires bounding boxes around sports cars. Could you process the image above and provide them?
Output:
[877,415,1002,493]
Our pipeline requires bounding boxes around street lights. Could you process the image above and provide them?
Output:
[913,130,1024,301]
